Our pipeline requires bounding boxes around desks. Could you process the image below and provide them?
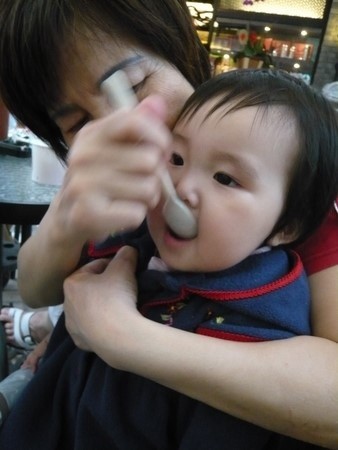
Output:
[0,146,62,243]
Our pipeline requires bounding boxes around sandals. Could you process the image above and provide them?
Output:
[1,304,63,350]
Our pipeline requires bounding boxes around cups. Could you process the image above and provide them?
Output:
[30,141,68,186]
[321,80,338,126]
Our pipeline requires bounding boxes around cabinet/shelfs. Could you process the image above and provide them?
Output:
[208,0,333,87]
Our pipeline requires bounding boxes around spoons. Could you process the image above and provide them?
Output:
[100,69,199,240]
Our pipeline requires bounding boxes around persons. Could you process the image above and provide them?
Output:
[0,0,337,450]
[1,300,64,353]
[0,67,338,450]
